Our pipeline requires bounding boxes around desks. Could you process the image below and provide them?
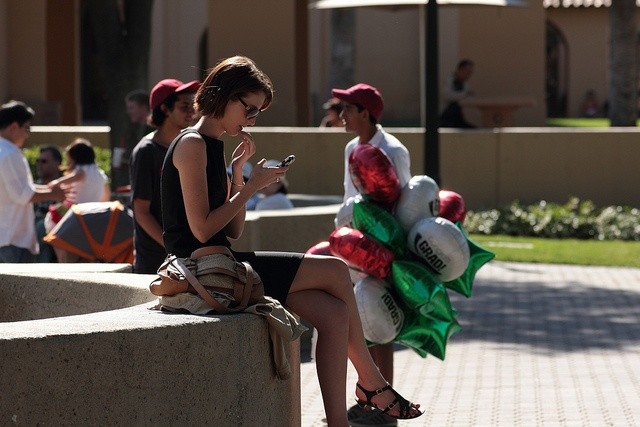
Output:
[448,96,535,129]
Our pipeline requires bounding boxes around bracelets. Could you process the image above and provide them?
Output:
[230,180,246,187]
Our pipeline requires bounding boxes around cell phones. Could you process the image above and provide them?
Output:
[278,154,296,168]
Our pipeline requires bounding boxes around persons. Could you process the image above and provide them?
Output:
[0,100,78,263]
[321,81,412,427]
[439,58,476,128]
[42,138,112,262]
[321,97,346,127]
[30,148,63,263]
[227,159,256,211]
[126,91,157,142]
[255,159,294,210]
[159,55,426,427]
[130,79,199,274]
[580,89,600,118]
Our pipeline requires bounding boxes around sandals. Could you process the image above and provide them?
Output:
[353,377,427,419]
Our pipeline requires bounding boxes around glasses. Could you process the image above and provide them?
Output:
[236,95,263,120]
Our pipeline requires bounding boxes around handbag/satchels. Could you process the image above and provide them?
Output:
[149,246,268,314]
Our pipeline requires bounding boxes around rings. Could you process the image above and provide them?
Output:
[276,177,279,183]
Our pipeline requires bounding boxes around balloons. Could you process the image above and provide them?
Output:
[443,221,496,298]
[397,307,460,361]
[328,225,395,278]
[350,199,407,258]
[348,144,401,204]
[353,275,406,345]
[407,216,469,282]
[390,260,455,323]
[392,175,440,233]
[438,190,466,221]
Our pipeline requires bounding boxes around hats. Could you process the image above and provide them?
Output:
[149,78,199,112]
[331,83,385,122]
